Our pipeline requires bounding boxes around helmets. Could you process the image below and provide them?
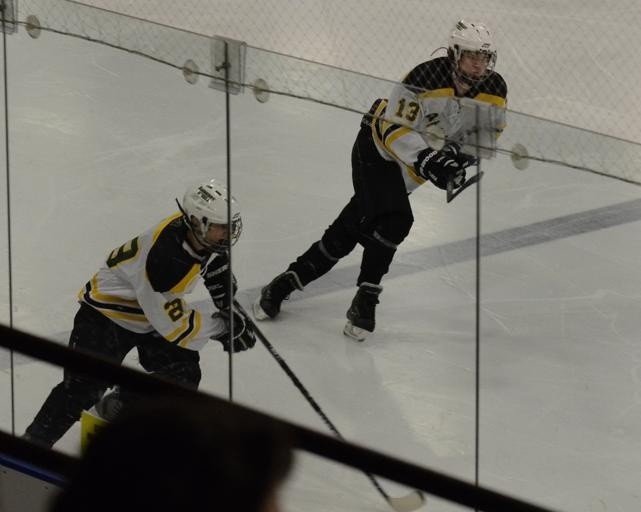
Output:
[445,18,501,86]
[182,179,242,256]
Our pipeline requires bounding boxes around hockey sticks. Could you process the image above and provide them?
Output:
[446,177,484,204]
[233,297,424,512]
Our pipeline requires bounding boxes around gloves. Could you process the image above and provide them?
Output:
[414,147,466,190]
[210,301,257,355]
[441,143,476,177]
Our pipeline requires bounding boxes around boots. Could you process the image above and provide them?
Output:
[345,281,382,331]
[259,262,304,317]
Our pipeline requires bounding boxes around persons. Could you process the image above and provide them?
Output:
[22,178,258,450]
[41,383,296,512]
[260,16,510,332]
